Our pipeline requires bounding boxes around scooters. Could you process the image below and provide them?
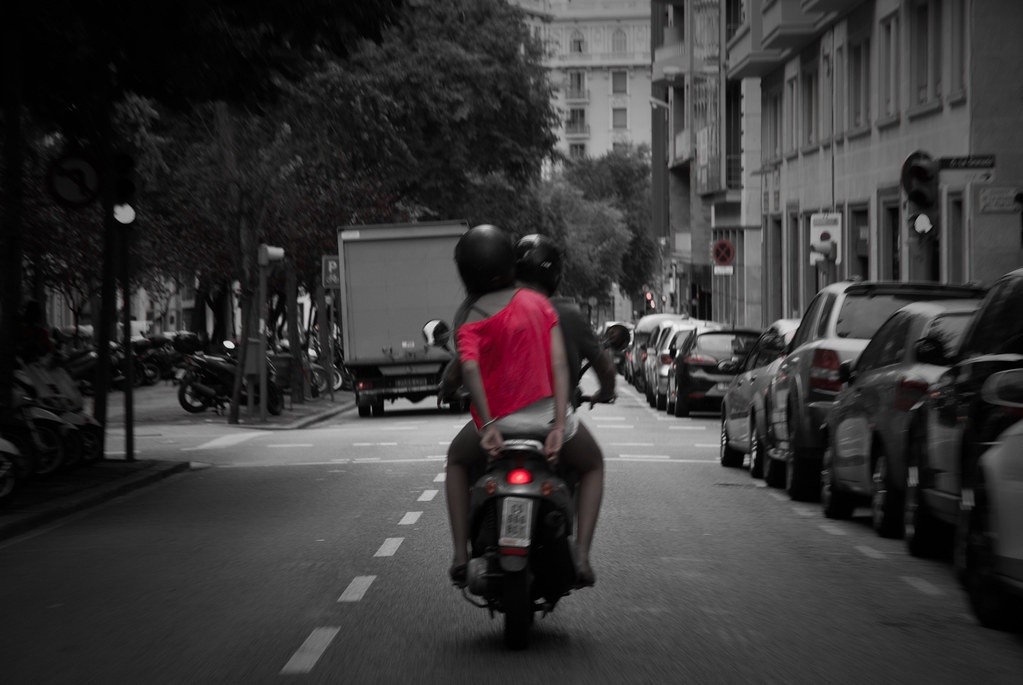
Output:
[421,318,632,650]
[0,320,355,516]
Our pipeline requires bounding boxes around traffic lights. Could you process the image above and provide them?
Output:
[645,291,656,311]
[258,242,286,267]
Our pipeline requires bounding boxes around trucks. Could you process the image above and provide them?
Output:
[335,219,475,420]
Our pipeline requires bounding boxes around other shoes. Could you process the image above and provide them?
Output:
[577,559,595,585]
[449,565,467,588]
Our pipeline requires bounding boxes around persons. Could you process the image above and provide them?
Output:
[453,235,616,411]
[446,223,606,588]
[434,322,450,346]
[18,301,57,359]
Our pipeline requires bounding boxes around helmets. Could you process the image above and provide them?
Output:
[454,225,516,295]
[512,234,562,296]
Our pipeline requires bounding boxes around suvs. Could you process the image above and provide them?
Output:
[760,278,986,503]
[897,266,1023,591]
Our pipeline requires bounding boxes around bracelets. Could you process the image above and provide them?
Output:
[555,427,566,433]
[482,418,497,428]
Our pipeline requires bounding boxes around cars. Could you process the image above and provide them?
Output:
[717,317,802,480]
[810,297,987,540]
[664,326,766,418]
[958,364,1023,634]
[592,311,726,411]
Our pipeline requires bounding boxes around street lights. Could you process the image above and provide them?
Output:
[108,197,136,466]
